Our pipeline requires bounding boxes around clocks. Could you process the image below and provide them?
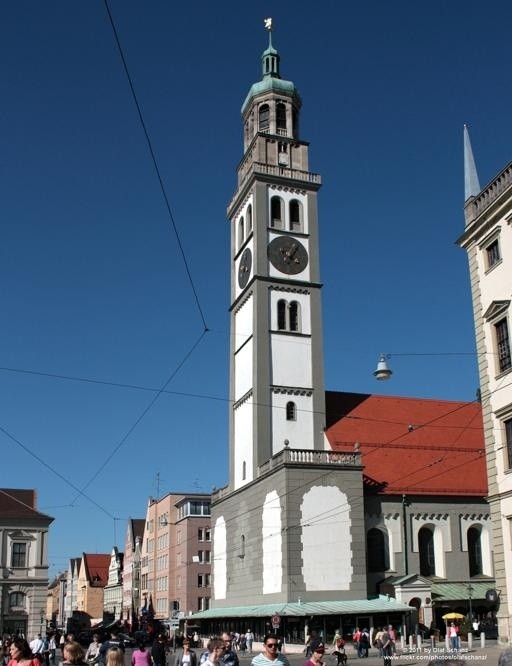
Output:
[239,248,251,289]
[268,236,308,274]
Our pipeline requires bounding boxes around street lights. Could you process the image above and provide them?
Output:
[466,583,474,631]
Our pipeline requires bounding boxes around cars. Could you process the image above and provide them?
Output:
[101,633,137,647]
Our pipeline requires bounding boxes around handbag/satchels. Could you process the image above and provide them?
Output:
[32,653,43,663]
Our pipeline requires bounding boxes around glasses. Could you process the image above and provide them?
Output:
[218,646,226,651]
[266,644,278,648]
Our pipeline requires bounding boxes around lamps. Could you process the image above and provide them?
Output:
[372,352,498,380]
[160,517,176,526]
[486,589,501,602]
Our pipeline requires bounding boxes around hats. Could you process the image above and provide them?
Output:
[311,640,328,651]
